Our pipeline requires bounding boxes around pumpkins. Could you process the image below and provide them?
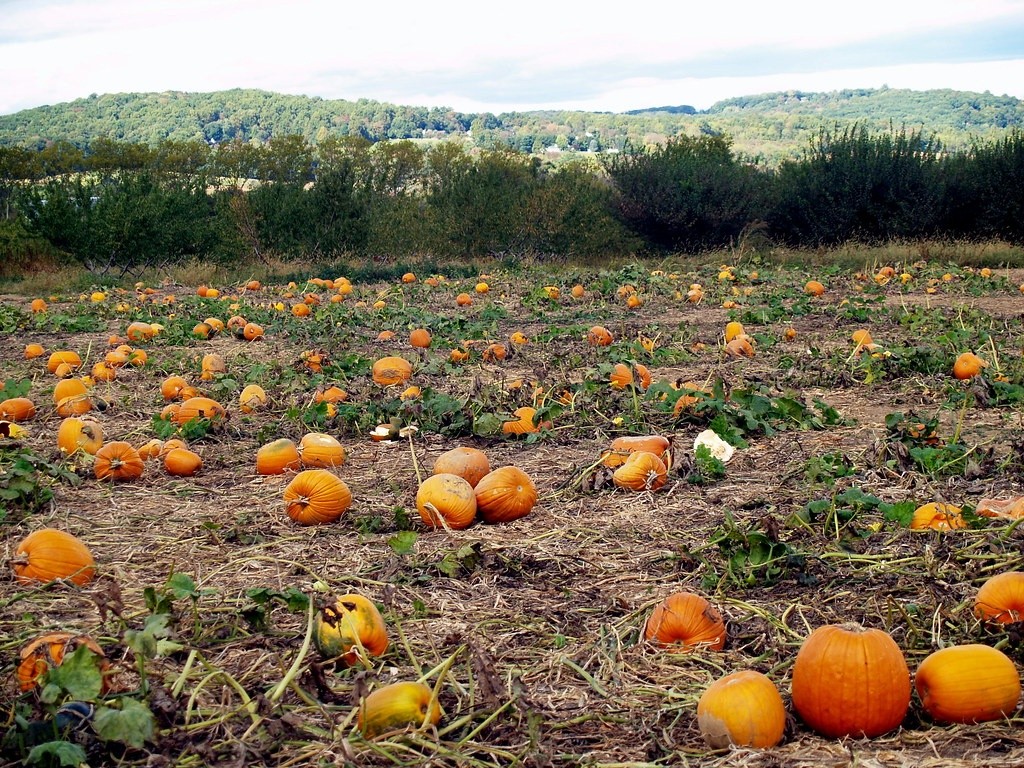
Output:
[0,264,1024,753]
